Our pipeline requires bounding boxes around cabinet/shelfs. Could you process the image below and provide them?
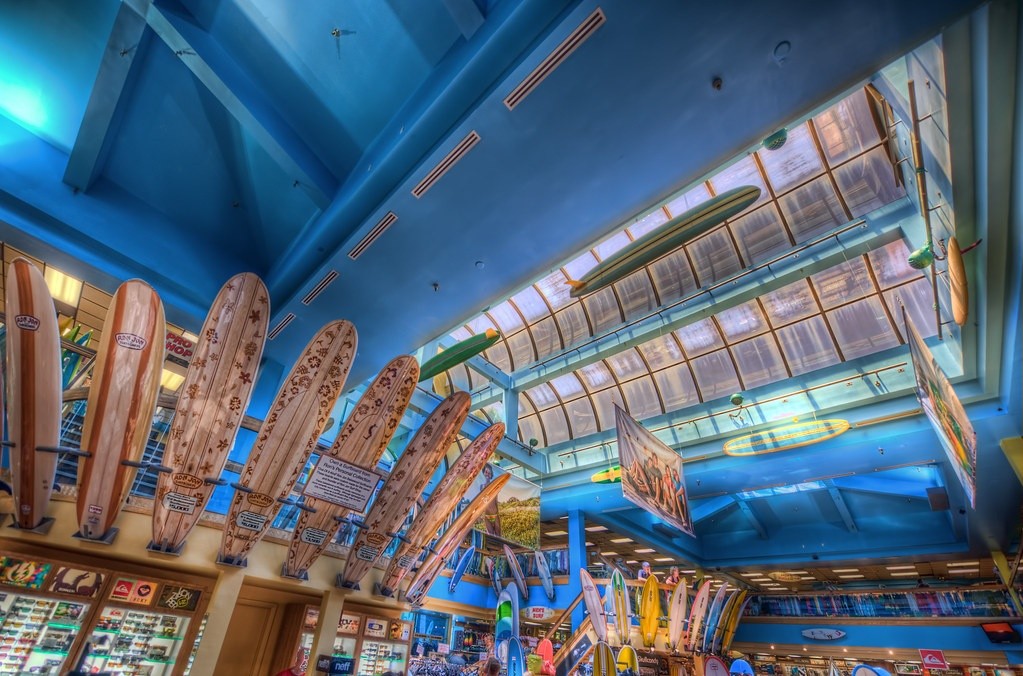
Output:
[0,548,210,675]
[269,600,413,676]
[723,648,1023,676]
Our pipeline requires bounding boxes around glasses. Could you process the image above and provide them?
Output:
[391,628,399,632]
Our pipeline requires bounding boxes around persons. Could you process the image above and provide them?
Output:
[635,452,665,509]
[636,561,659,615]
[627,450,653,488]
[915,577,930,588]
[478,462,502,537]
[478,655,502,676]
[660,464,679,519]
[663,565,682,611]
[672,469,690,529]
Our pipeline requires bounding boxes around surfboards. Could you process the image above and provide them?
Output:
[342,390,472,589]
[829,656,841,676]
[580,568,607,641]
[723,419,850,458]
[852,664,879,676]
[417,326,501,381]
[504,544,528,600]
[536,638,553,670]
[7,256,62,529]
[802,629,846,640]
[612,567,632,644]
[565,185,762,298]
[507,636,525,676]
[704,655,728,676]
[380,422,506,592]
[947,235,966,326]
[484,558,501,598]
[219,320,359,564]
[591,465,620,484]
[404,473,511,603]
[721,588,747,656]
[687,580,711,650]
[152,273,270,548]
[731,659,754,675]
[449,546,475,591]
[535,550,554,600]
[712,589,738,654]
[616,646,640,675]
[640,576,661,647]
[494,591,512,676]
[668,577,687,650]
[593,643,615,676]
[286,352,421,576]
[703,580,728,651]
[75,278,166,538]
